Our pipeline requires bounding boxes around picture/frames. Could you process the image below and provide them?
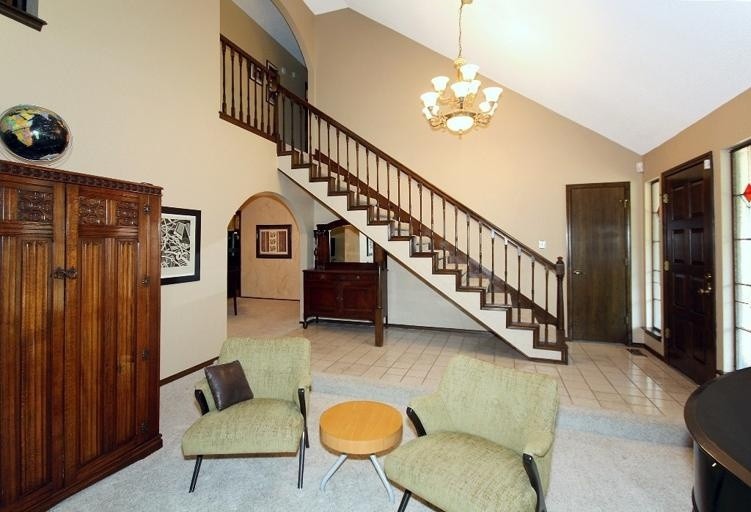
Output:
[249,61,263,85]
[266,60,280,106]
[256,225,292,259]
[161,208,200,284]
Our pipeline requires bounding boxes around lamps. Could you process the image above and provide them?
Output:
[418,0,504,138]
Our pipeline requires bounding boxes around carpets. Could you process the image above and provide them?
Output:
[48,370,695,512]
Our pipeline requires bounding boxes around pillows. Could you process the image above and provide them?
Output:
[204,360,253,411]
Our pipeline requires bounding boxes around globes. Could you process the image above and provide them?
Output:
[0,105,73,169]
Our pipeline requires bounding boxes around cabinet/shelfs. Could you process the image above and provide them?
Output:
[302,269,387,347]
[0,160,162,512]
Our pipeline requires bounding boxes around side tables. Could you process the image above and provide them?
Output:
[319,401,403,506]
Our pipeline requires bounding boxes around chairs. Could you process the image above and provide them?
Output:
[180,337,311,492]
[383,356,559,512]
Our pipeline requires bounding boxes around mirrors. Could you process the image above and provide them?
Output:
[317,220,387,269]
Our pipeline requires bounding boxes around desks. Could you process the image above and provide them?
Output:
[685,367,751,512]
